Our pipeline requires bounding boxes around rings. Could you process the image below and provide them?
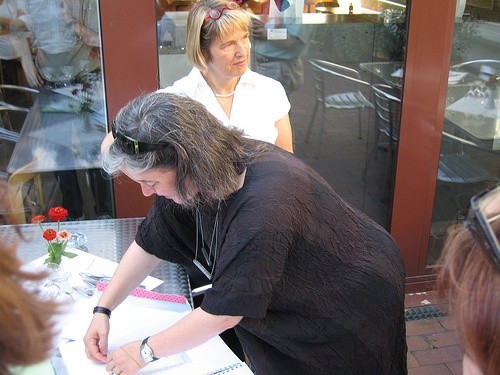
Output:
[112,367,118,375]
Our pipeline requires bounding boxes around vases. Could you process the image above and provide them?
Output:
[484,87,496,110]
[79,111,94,133]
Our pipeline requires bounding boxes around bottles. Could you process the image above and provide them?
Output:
[349,3,353,13]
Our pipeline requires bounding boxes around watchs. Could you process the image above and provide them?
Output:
[141,335,160,362]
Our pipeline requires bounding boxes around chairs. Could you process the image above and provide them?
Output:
[307,59,376,148]
[0,85,59,223]
[451,59,500,151]
[360,81,497,223]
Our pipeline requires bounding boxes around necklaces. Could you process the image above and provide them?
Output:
[214,92,235,97]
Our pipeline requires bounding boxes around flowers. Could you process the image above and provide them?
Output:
[73,82,100,117]
[31,206,76,267]
[483,71,500,90]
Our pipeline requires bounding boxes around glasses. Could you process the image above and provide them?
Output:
[466,181,500,273]
[112,120,170,156]
[205,2,239,21]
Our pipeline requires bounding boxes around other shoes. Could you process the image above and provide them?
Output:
[67,214,85,221]
[98,215,112,219]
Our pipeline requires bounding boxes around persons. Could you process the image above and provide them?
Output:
[0,0,115,221]
[436,184,500,375]
[83,92,406,375]
[162,0,293,153]
[241,0,309,103]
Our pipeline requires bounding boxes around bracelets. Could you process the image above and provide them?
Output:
[93,306,111,317]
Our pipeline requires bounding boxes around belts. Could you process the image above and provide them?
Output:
[39,68,100,89]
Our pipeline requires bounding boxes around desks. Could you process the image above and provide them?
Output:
[317,6,383,20]
[0,217,194,375]
[443,92,500,201]
[359,60,483,160]
[7,83,112,221]
